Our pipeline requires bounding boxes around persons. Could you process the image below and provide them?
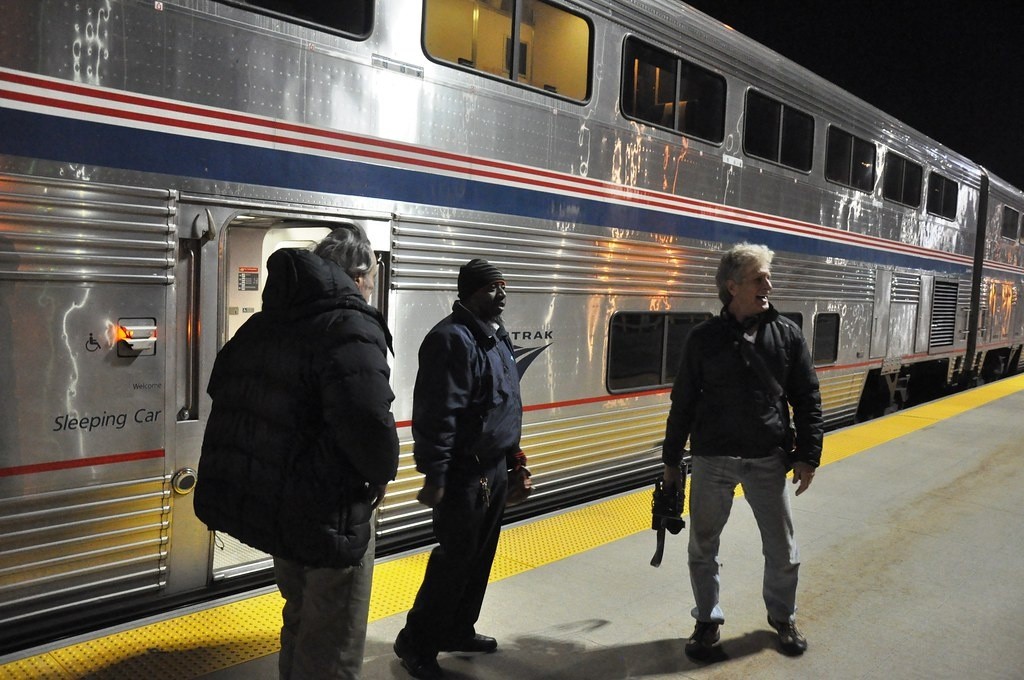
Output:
[663,243,824,661]
[194,224,399,679]
[392,259,532,680]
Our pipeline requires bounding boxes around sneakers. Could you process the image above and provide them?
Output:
[685,619,720,656]
[768,612,807,653]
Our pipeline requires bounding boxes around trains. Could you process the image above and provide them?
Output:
[1,0,1024,656]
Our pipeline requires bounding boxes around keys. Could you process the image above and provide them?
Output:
[480,477,491,510]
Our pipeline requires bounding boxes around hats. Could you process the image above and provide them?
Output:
[458,259,506,303]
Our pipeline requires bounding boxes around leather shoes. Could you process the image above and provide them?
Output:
[440,634,497,651]
[393,643,443,680]
